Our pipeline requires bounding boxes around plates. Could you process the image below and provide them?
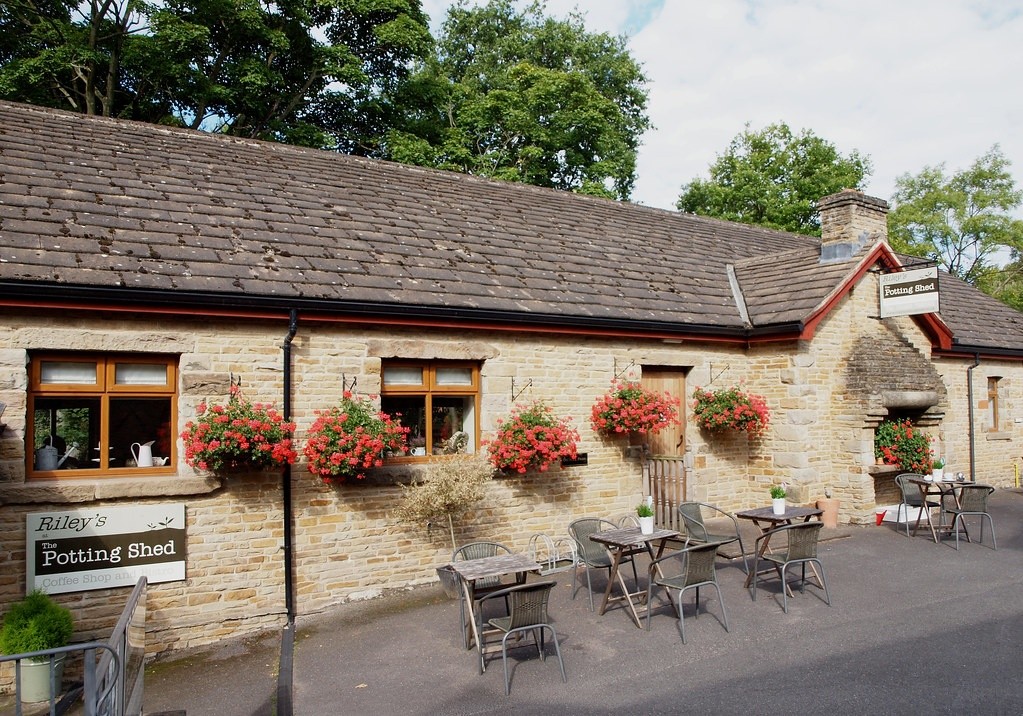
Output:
[943,479,956,481]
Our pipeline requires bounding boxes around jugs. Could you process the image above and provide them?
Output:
[131,440,155,467]
[35,434,80,470]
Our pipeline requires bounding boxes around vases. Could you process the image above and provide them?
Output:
[817,500,839,528]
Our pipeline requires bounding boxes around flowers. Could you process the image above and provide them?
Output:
[591,379,682,437]
[304,391,409,483]
[688,386,769,444]
[179,386,297,472]
[874,417,933,476]
[486,398,582,476]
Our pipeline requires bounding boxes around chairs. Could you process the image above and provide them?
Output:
[452,502,832,695]
[895,473,1000,552]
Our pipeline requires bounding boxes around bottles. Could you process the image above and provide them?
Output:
[956,472,964,482]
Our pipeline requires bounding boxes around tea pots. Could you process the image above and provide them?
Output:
[153,456,169,466]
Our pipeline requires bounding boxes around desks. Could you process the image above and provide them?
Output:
[449,553,546,671]
[735,507,826,600]
[908,479,977,544]
[588,527,680,628]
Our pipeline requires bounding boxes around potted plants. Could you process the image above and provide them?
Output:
[0,587,74,703]
[769,488,785,514]
[395,448,495,598]
[636,506,655,535]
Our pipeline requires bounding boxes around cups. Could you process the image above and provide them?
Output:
[410,446,425,456]
[944,472,954,479]
